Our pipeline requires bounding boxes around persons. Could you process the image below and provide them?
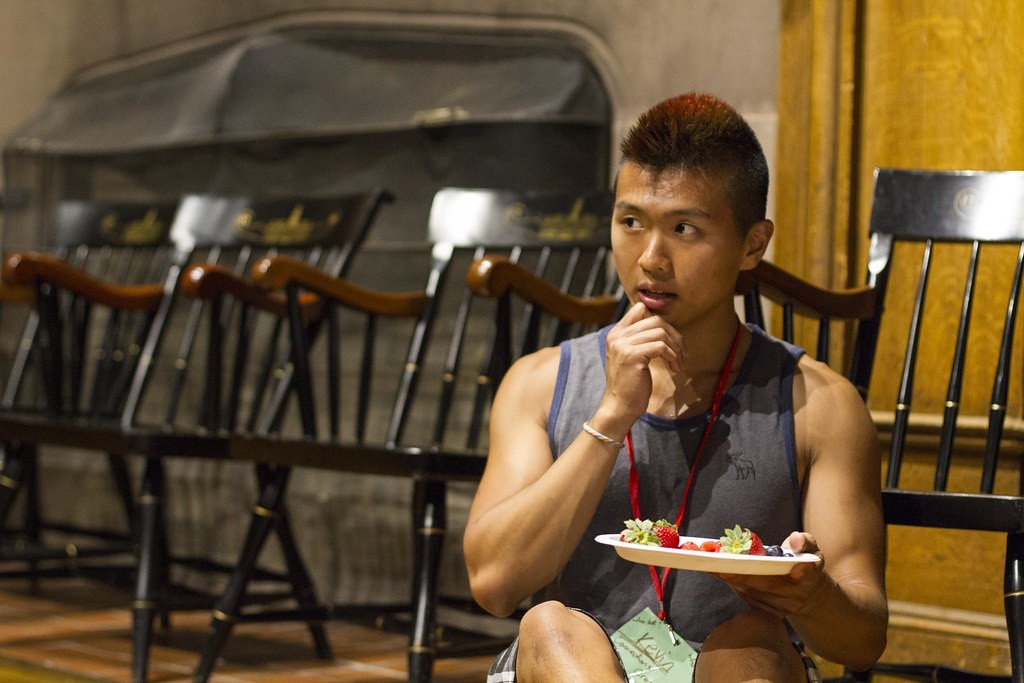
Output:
[461,91,891,682]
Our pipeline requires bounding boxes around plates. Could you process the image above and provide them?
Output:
[595,534,820,577]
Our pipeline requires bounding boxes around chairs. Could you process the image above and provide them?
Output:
[5,191,185,630]
[732,162,1024,683]
[191,182,631,683]
[2,190,397,683]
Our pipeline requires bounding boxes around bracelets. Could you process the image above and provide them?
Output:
[582,421,629,451]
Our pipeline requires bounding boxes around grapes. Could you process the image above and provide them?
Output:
[767,545,795,557]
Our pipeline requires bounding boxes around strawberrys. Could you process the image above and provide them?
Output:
[620,518,766,556]
